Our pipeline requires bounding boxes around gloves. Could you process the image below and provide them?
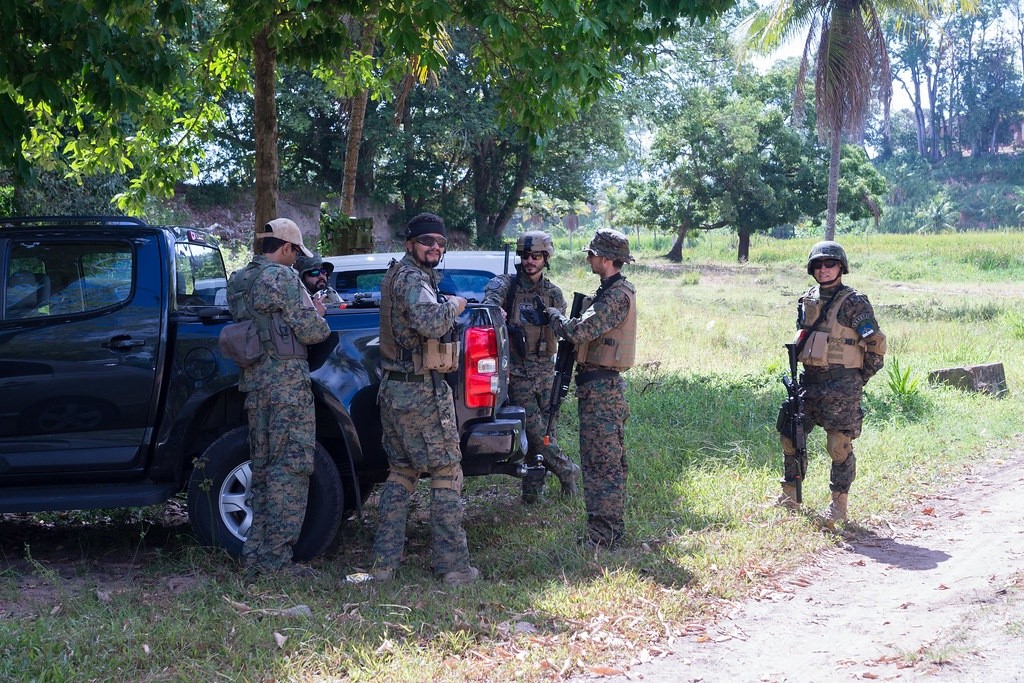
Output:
[520,296,552,327]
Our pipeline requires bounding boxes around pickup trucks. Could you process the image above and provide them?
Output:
[0,216,545,571]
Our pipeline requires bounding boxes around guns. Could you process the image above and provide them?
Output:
[506,324,529,359]
[328,291,380,308]
[542,289,594,447]
[779,343,808,504]
[503,242,510,274]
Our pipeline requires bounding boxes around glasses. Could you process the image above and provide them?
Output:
[307,268,327,278]
[521,253,544,261]
[812,259,841,272]
[586,251,597,258]
[413,235,447,249]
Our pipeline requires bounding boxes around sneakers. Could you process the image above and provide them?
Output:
[558,465,581,501]
[375,569,392,583]
[444,567,478,584]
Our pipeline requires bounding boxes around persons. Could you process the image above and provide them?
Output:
[293,253,345,303]
[542,228,636,552]
[776,240,886,522]
[226,218,331,572]
[481,229,579,506]
[371,212,478,586]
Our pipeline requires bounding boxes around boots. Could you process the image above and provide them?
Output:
[774,484,798,510]
[822,492,847,528]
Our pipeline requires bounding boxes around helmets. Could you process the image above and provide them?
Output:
[516,232,553,255]
[807,242,849,275]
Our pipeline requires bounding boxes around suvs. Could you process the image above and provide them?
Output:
[229,251,520,303]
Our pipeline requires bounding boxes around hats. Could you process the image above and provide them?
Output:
[292,254,334,277]
[581,229,635,265]
[256,218,314,259]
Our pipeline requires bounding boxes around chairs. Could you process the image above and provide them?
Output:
[4,270,52,321]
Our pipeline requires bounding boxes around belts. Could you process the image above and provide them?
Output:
[383,370,445,382]
